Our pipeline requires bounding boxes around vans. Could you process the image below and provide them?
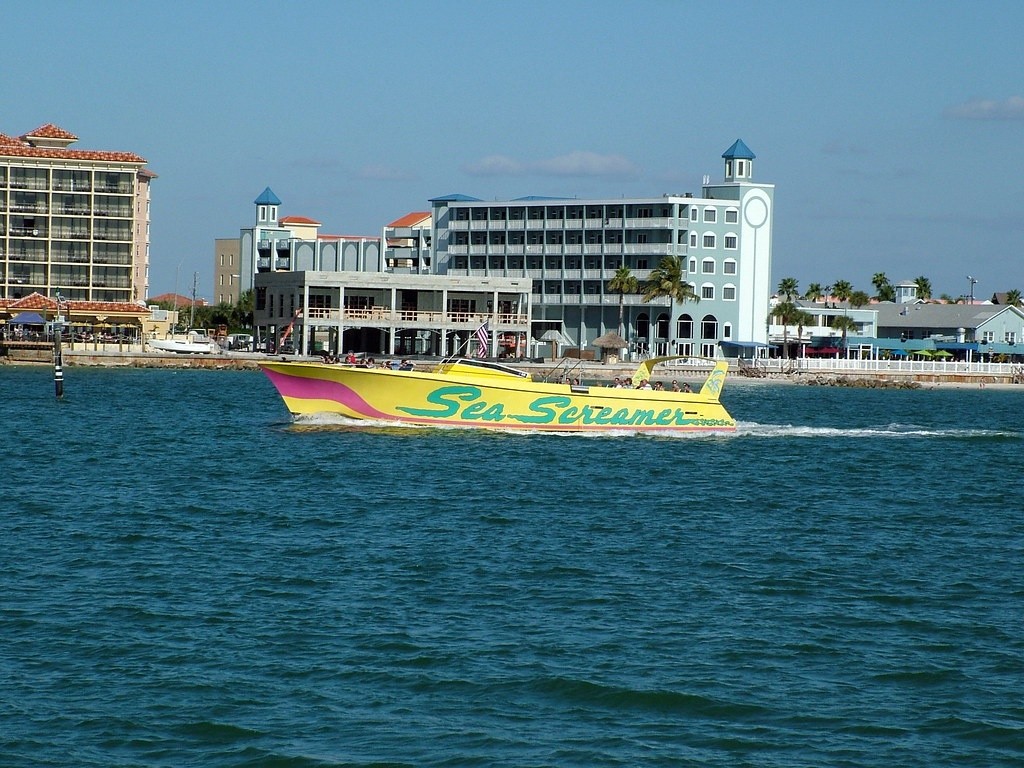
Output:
[226,333,253,347]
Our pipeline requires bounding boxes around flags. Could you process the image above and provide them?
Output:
[476,323,489,358]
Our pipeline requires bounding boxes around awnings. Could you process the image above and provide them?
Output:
[719,340,778,348]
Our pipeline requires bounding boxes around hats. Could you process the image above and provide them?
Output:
[349,350,354,355]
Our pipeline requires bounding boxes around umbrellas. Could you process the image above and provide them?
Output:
[871,350,954,360]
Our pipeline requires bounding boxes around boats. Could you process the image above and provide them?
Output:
[256,355,739,435]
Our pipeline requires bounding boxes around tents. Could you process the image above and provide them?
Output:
[5,314,45,326]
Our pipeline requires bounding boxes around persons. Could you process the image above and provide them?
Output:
[0,325,133,345]
[940,357,966,362]
[324,349,696,394]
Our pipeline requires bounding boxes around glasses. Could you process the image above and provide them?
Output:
[684,385,687,386]
[672,383,677,384]
